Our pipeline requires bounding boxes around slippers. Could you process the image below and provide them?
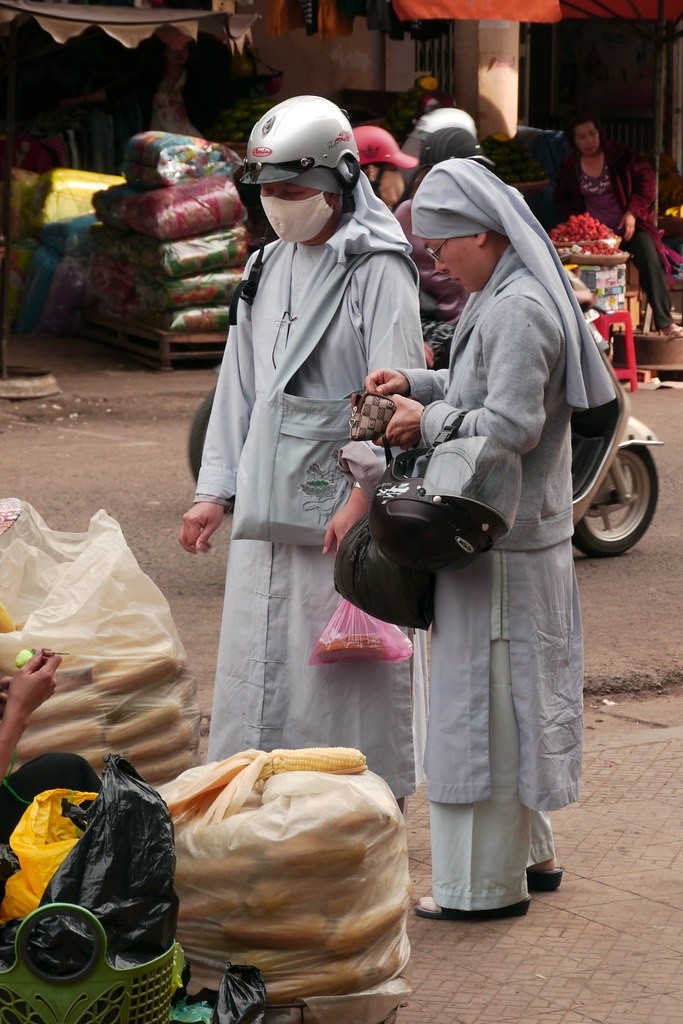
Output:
[526,868,563,891]
[414,895,530,919]
[668,327,683,340]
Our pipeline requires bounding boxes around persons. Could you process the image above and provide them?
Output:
[509,117,683,338]
[350,107,469,325]
[364,159,617,923]
[0,647,102,904]
[181,99,415,810]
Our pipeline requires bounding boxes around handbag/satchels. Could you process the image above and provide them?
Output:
[227,251,420,547]
[308,594,413,666]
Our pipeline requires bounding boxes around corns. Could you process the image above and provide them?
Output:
[176,746,408,1004]
[0,602,202,787]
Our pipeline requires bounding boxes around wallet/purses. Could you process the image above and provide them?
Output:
[349,392,397,443]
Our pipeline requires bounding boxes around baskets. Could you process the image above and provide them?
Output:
[0,902,175,1023]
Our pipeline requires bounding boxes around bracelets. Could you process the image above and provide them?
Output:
[352,481,361,488]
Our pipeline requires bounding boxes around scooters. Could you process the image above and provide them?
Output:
[187,269,665,558]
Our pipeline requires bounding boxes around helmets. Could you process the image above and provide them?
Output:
[407,107,477,143]
[419,127,495,168]
[368,436,523,574]
[237,95,361,186]
[350,126,419,169]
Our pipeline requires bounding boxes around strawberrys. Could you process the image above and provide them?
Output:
[550,212,623,256]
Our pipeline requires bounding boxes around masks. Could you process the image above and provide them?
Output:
[260,190,334,243]
[368,164,407,205]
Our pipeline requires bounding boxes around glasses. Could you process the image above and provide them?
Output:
[425,238,449,263]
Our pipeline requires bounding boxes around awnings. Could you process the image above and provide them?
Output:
[0,0,261,57]
